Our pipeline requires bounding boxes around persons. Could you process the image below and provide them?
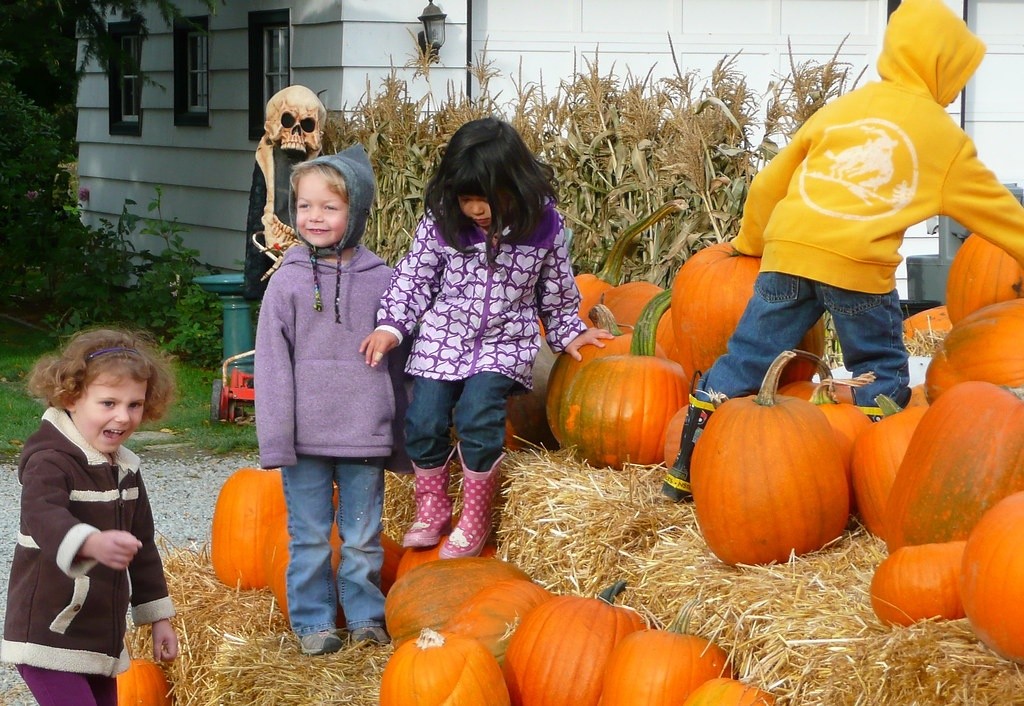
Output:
[359,118,616,561]
[253,144,397,654]
[1,327,180,706]
[659,0,1023,503]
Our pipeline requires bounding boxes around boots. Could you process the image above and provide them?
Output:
[851,403,886,425]
[440,440,506,564]
[403,447,456,547]
[659,371,720,504]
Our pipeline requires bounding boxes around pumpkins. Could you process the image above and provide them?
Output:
[505,229,1024,671]
[214,465,785,706]
[116,657,175,706]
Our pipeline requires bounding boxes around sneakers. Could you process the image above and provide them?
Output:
[352,625,393,646]
[301,630,344,654]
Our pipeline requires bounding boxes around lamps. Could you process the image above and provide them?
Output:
[418,0,447,64]
